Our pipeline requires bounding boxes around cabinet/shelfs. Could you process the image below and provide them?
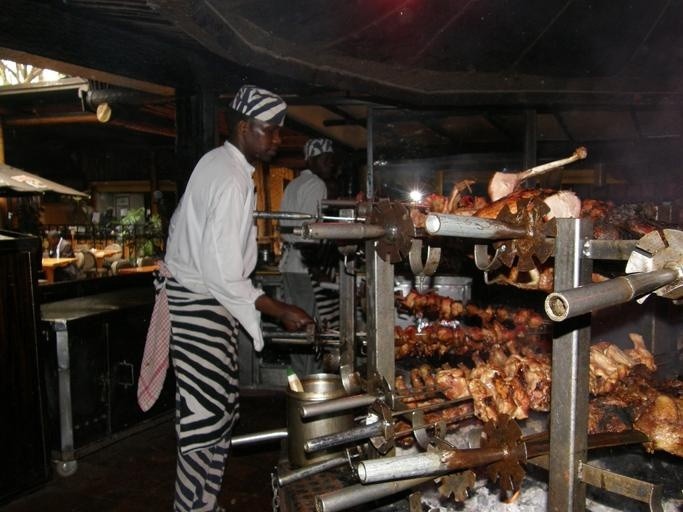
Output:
[41,283,188,478]
[1,228,56,507]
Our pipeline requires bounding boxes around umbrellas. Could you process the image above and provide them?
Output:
[1,162,91,229]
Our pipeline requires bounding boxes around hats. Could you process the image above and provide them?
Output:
[302,139,333,160]
[230,85,287,126]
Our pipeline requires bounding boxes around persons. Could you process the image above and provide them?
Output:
[47,229,80,282]
[276,137,338,377]
[152,85,318,512]
[97,209,118,239]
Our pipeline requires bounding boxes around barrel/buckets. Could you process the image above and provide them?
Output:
[287,372,357,471]
[434,274,473,305]
